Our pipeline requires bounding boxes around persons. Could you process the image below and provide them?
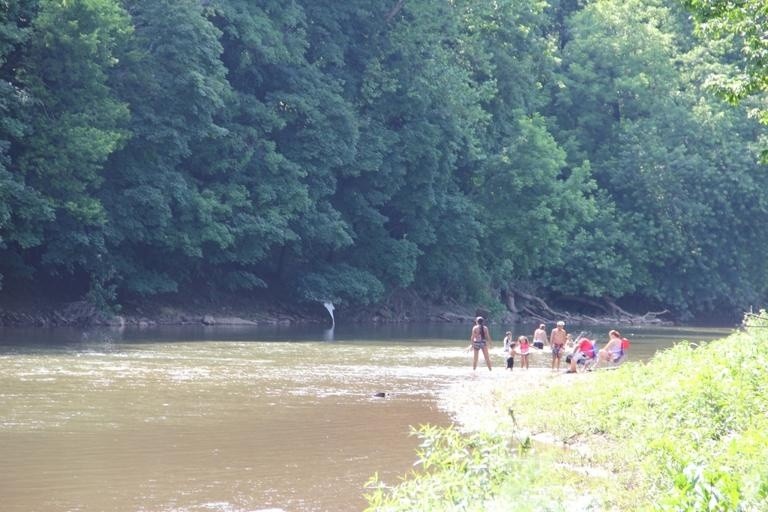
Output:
[551,321,568,371]
[503,331,513,352]
[566,331,595,374]
[515,335,539,369]
[532,323,549,349]
[587,329,622,371]
[470,316,492,371]
[566,334,574,348]
[505,342,529,371]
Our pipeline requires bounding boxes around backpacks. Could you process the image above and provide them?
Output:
[622,338,630,348]
